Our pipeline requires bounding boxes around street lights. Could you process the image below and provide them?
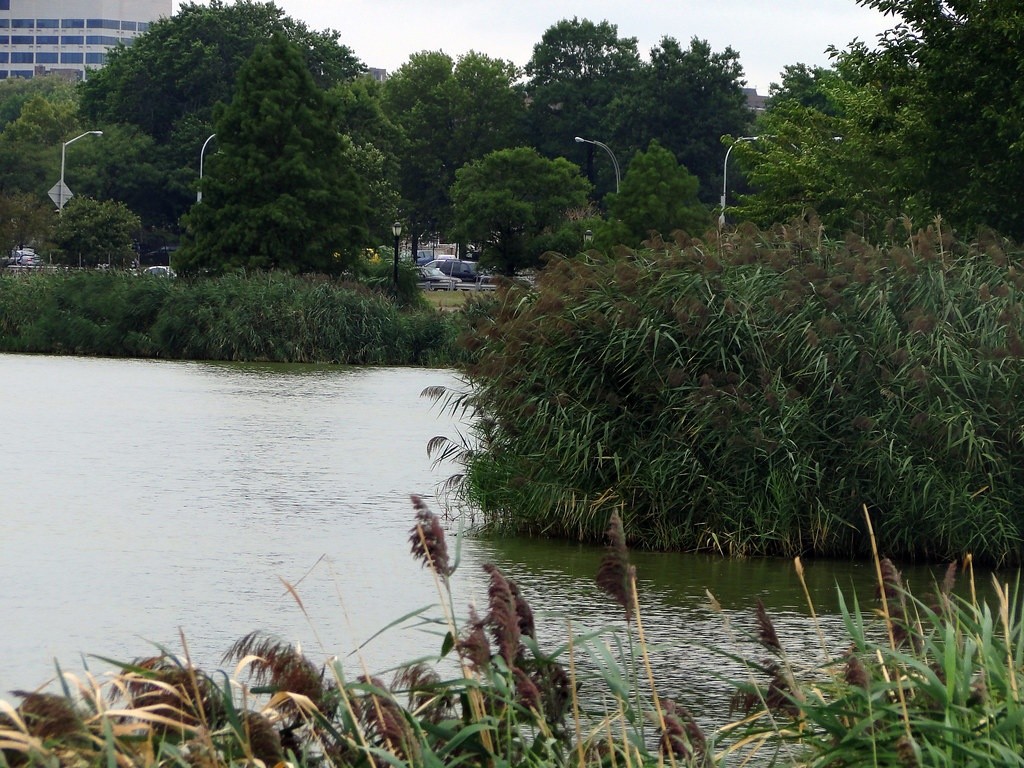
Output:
[574,136,621,194]
[59,130,104,217]
[721,137,761,225]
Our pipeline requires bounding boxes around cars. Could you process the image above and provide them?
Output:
[425,255,457,269]
[441,259,499,285]
[409,266,462,291]
[416,250,440,266]
[335,246,380,265]
[135,265,177,281]
[2,251,41,266]
